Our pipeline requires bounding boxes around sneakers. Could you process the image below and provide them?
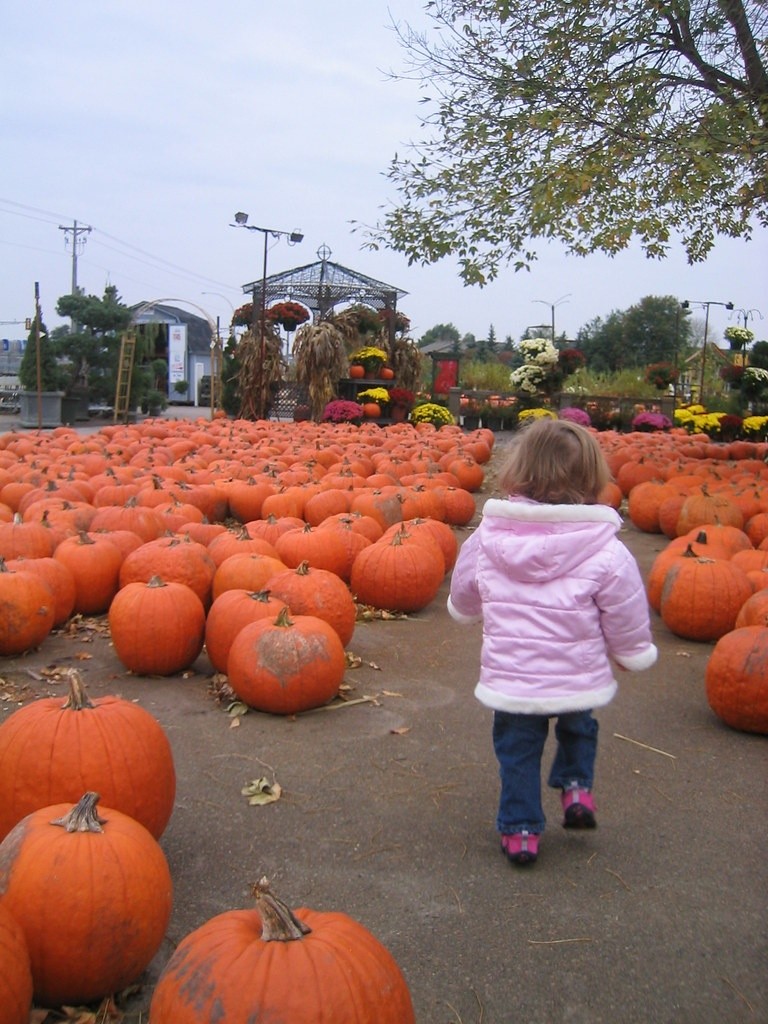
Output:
[562,780,597,830]
[502,830,541,865]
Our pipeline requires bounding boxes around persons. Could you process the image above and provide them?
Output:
[446,418,659,868]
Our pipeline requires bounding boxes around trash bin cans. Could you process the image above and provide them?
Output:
[61,397,81,428]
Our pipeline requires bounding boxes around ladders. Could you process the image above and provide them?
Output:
[113,333,137,424]
[210,336,223,420]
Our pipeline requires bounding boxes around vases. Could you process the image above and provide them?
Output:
[392,404,406,420]
[729,380,740,389]
[247,321,253,330]
[365,369,377,379]
[284,321,296,330]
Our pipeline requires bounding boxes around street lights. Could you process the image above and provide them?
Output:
[668,304,692,424]
[226,212,303,418]
[681,300,734,406]
[727,306,764,375]
[34,280,41,435]
[531,293,572,370]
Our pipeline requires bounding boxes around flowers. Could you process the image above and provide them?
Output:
[632,401,768,443]
[723,326,754,343]
[232,303,254,326]
[349,346,387,368]
[719,365,768,395]
[357,387,455,428]
[323,399,364,423]
[267,302,309,325]
[510,338,591,428]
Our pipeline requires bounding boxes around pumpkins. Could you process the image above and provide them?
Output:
[364,402,381,419]
[0,897,34,1024]
[350,364,364,378]
[0,668,177,842]
[0,793,174,1005]
[379,368,394,379]
[0,408,496,714]
[149,875,416,1024]
[416,392,663,422]
[581,429,768,736]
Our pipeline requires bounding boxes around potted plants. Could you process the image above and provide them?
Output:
[16,315,65,427]
[644,361,678,389]
[730,341,742,350]
